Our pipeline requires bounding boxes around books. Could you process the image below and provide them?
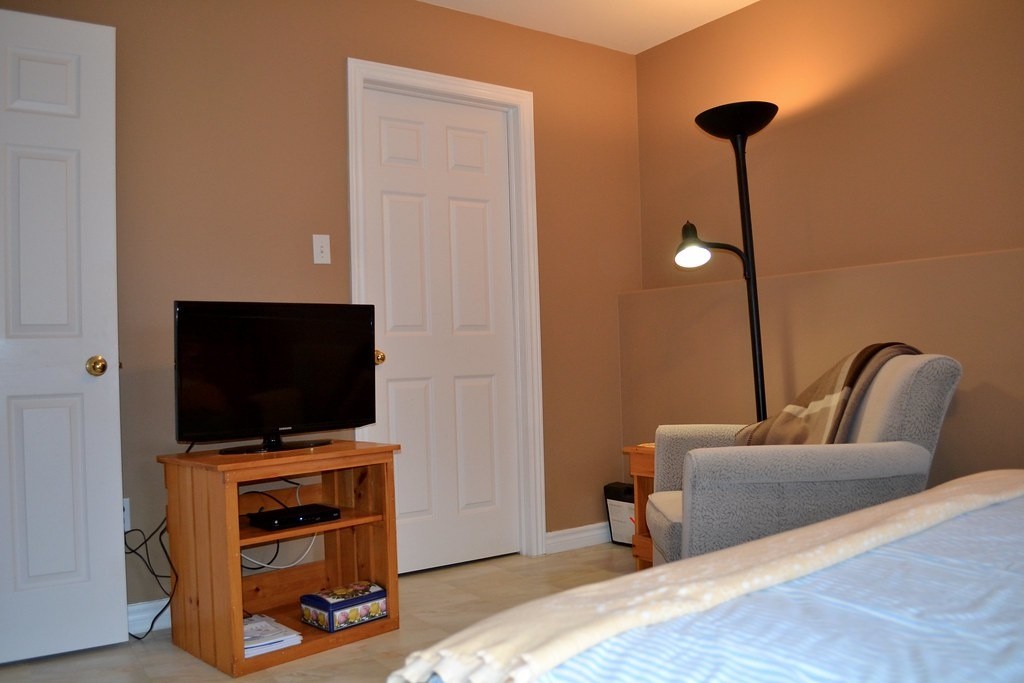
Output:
[243,613,304,658]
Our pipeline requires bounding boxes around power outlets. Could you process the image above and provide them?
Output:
[313,234,331,264]
[123,498,131,532]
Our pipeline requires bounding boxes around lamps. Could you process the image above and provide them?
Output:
[673,99,778,422]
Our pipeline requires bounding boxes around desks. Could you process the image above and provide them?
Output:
[622,444,655,571]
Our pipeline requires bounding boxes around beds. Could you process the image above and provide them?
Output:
[381,466,1024,683]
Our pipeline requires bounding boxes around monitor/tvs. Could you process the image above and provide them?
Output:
[173,299,379,455]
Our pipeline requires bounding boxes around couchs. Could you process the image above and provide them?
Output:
[646,354,962,565]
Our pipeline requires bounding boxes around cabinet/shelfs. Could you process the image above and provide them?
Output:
[156,438,399,674]
